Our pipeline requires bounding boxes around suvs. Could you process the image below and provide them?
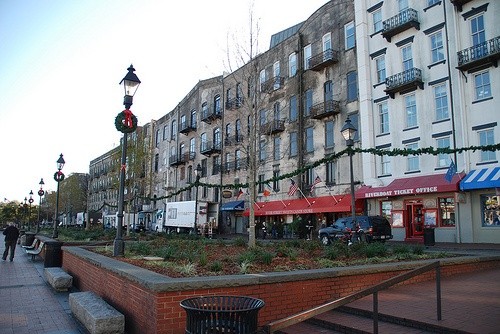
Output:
[318,215,394,246]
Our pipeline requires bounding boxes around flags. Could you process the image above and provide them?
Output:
[444,162,457,184]
[236,175,323,200]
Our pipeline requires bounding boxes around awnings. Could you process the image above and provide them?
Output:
[357,167,500,199]
[223,193,364,215]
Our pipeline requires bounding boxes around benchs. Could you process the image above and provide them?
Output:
[26,242,44,261]
[22,238,38,250]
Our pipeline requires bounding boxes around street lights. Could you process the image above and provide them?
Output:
[194,163,203,236]
[133,183,139,232]
[113,64,142,257]
[52,153,66,238]
[340,117,358,243]
[28,190,33,231]
[17,197,27,229]
[36,178,45,233]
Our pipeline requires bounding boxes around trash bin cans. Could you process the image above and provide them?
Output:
[179,295,266,333]
[423,228,435,245]
[44,241,63,268]
[20,230,25,236]
[25,233,37,250]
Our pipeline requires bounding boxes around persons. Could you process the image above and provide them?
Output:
[1,222,19,262]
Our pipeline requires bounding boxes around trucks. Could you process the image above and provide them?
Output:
[155,200,219,239]
